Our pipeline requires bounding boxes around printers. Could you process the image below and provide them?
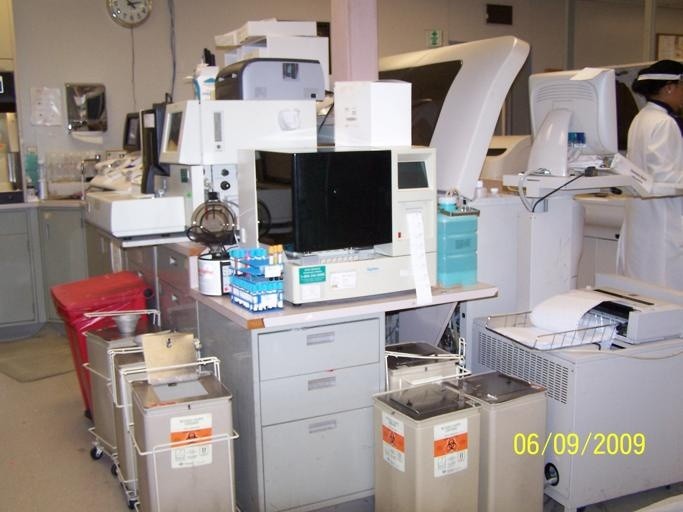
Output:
[472,270,683,512]
[214,59,324,100]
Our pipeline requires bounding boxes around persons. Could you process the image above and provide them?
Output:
[615,59,683,291]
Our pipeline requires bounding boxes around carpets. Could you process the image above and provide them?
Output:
[0,322,76,383]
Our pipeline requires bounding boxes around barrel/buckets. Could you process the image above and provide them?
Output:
[437,197,481,287]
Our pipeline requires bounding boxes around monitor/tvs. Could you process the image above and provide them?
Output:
[121,113,140,151]
[159,100,203,166]
[498,66,634,190]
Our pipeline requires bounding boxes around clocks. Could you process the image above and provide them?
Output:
[106,1,152,29]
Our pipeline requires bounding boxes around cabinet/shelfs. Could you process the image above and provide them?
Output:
[0,207,40,327]
[40,208,90,323]
[121,246,156,283]
[87,228,115,279]
[157,246,194,332]
[196,301,387,512]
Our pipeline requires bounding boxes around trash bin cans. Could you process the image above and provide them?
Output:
[50,271,154,419]
